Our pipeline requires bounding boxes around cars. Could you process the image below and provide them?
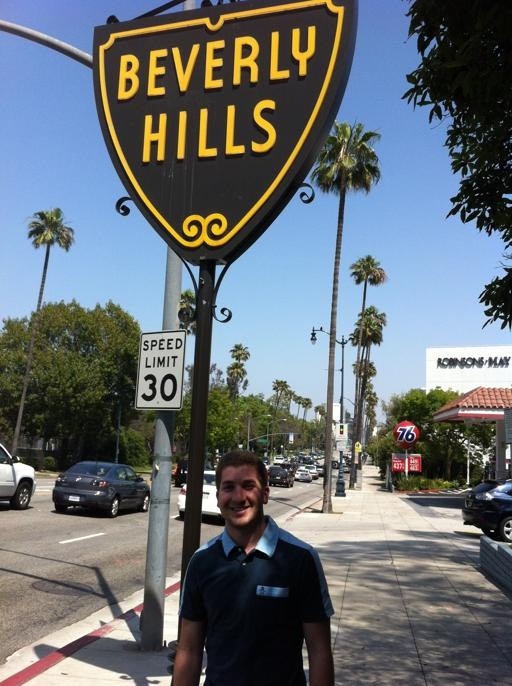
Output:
[53,461,150,518]
[0,443,36,510]
[462,479,512,543]
[175,460,222,526]
[262,452,351,488]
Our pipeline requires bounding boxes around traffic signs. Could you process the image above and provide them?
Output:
[133,328,187,411]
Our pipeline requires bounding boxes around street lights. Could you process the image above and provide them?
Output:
[311,326,354,496]
[222,414,286,458]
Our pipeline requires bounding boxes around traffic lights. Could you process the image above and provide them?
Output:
[340,425,343,434]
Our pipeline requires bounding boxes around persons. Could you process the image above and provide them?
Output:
[172,449,335,685]
